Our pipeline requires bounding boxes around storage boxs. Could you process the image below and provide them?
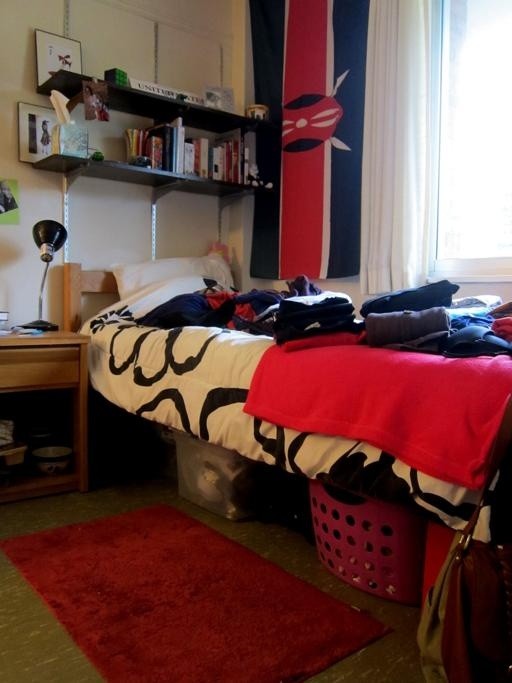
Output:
[172,429,266,522]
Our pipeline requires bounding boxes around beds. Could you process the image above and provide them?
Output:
[63,263,512,526]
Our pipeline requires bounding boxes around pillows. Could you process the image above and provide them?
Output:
[114,252,233,300]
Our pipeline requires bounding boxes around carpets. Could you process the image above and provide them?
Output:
[2,502,393,681]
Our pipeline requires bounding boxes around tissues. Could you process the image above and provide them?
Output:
[49,90,88,160]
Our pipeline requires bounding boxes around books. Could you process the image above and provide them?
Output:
[145,117,249,185]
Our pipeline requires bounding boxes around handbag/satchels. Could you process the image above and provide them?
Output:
[416,441,512,683]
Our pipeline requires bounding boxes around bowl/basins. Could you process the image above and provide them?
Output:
[33,446,73,475]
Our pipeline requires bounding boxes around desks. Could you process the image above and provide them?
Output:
[0,332,91,505]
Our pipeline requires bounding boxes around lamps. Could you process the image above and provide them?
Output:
[19,220,68,330]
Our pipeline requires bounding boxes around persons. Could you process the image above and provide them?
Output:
[40,119,51,156]
[85,86,110,122]
[64,55,72,67]
[0,179,18,213]
[58,56,65,65]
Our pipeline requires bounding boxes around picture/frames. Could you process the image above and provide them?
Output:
[16,103,60,164]
[35,28,82,88]
